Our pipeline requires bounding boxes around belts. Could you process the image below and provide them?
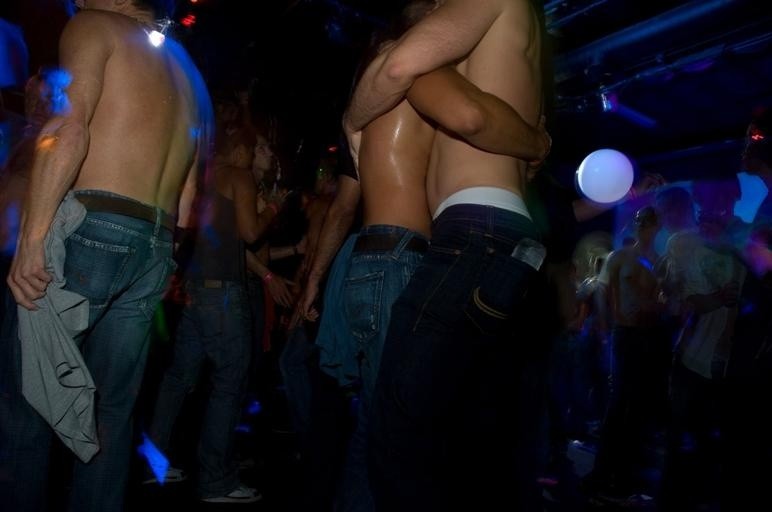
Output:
[353,234,428,255]
[75,194,175,232]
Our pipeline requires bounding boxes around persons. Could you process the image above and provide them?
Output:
[1,0,771,512]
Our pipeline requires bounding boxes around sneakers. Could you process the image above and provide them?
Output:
[143,464,187,484]
[200,486,262,503]
